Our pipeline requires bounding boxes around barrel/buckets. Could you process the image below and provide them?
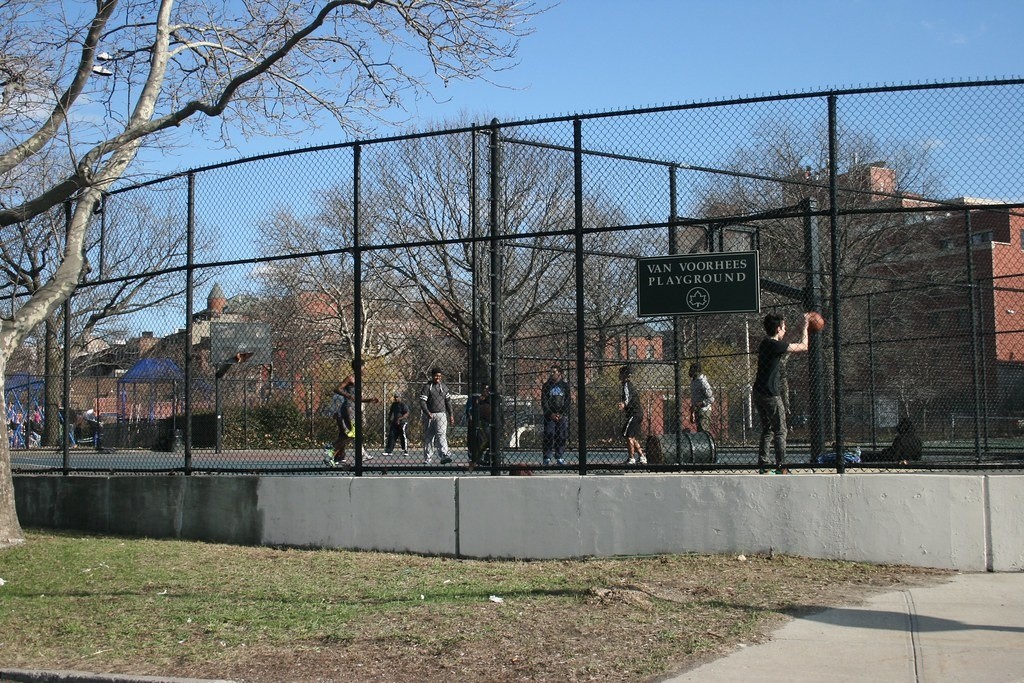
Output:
[646,431,716,465]
[167,428,183,452]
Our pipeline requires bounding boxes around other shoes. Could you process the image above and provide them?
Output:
[774,463,792,475]
[637,456,648,464]
[542,458,553,464]
[362,454,374,460]
[381,452,393,456]
[440,456,452,464]
[759,468,776,475]
[556,458,567,465]
[620,458,636,467]
[403,453,409,456]
[322,454,335,468]
[423,457,431,464]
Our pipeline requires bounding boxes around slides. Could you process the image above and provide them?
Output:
[76,415,104,444]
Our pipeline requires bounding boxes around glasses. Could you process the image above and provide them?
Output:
[394,396,398,398]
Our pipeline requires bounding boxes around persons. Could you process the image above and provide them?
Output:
[688,363,715,431]
[752,312,811,476]
[467,384,508,465]
[541,365,570,465]
[7,401,106,450]
[832,418,923,463]
[420,368,454,464]
[381,393,409,455]
[330,359,379,464]
[617,367,648,463]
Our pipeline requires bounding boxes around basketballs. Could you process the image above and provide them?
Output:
[808,311,825,331]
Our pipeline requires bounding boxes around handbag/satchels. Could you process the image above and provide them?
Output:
[342,419,356,438]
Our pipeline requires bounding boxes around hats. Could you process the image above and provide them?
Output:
[432,367,441,373]
[394,393,400,396]
